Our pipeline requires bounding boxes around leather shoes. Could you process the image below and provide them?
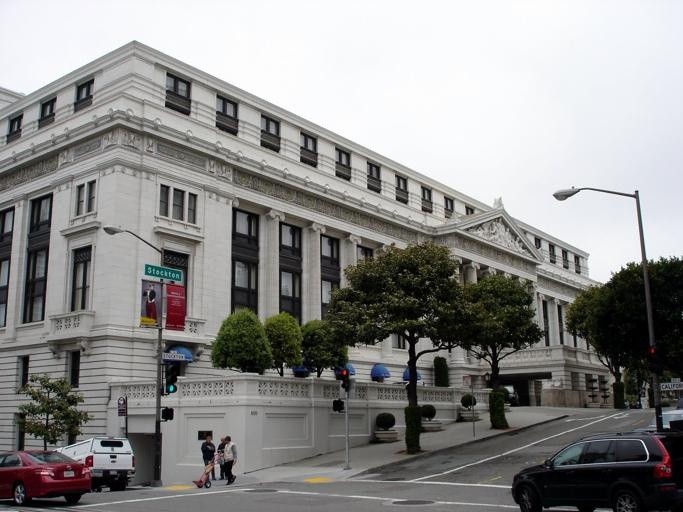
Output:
[226,475,236,485]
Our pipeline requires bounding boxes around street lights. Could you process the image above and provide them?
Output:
[341,369,350,392]
[552,186,663,432]
[103,227,164,487]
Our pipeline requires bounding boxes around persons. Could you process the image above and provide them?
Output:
[142,281,158,323]
[217,436,226,480]
[201,434,216,480]
[223,435,236,484]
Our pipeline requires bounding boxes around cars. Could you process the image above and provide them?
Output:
[511,429,683,512]
[0,450,92,504]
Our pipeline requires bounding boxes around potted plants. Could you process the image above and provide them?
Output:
[459,395,479,421]
[373,413,399,442]
[600,380,613,408]
[421,404,442,431]
[587,378,601,408]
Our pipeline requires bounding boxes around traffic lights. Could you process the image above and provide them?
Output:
[165,363,178,393]
[648,344,657,374]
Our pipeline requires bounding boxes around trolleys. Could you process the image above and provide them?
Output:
[192,453,221,488]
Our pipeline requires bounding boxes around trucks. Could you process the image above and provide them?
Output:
[50,436,136,492]
